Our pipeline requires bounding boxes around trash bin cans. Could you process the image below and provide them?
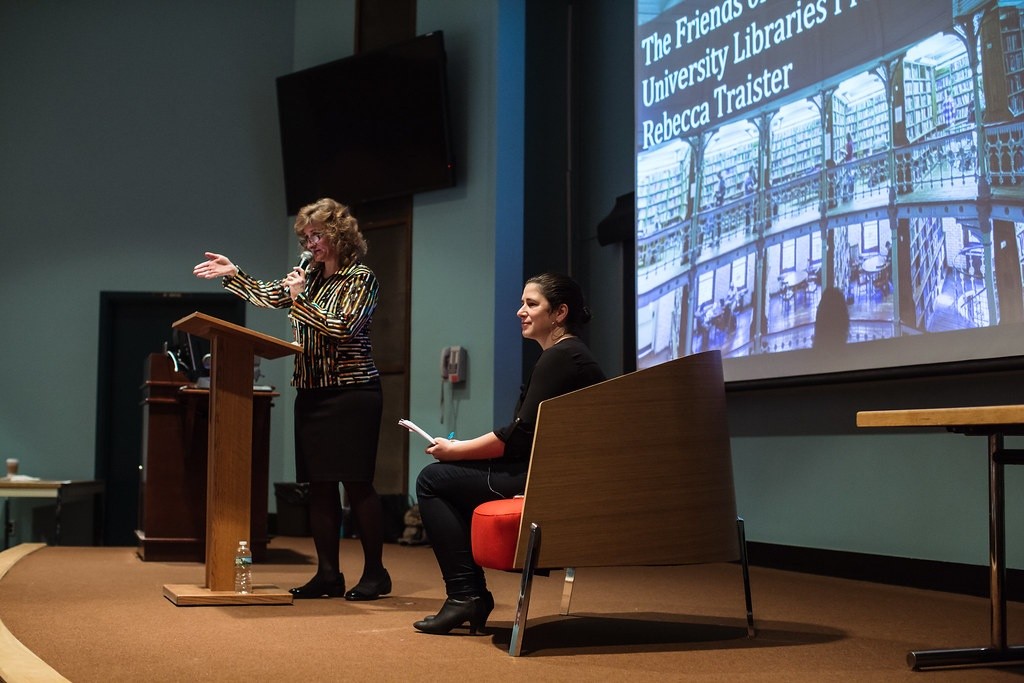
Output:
[274,482,311,536]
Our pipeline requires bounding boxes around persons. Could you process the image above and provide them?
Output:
[413,275,606,634]
[193,198,393,601]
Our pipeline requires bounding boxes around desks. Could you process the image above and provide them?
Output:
[0,479,71,552]
[856,404,1024,669]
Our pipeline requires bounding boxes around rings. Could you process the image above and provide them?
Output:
[291,277,294,281]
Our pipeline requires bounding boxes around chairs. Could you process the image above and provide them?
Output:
[471,350,756,658]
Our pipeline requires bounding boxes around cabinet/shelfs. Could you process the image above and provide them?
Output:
[135,352,280,561]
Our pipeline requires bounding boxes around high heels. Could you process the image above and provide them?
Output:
[424,591,494,632]
[413,596,486,636]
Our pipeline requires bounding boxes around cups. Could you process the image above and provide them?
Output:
[7,459,19,478]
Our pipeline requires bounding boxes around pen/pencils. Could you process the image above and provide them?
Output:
[447,431,455,439]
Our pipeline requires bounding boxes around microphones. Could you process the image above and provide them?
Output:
[284,251,313,293]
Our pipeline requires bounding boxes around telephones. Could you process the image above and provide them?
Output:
[440,345,467,383]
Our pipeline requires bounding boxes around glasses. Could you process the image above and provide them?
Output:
[299,233,324,249]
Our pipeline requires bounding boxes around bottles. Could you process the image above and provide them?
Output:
[234,540,253,595]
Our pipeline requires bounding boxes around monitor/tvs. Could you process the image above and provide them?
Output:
[275,30,455,216]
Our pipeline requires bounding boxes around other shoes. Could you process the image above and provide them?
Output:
[346,568,392,601]
[288,573,345,597]
